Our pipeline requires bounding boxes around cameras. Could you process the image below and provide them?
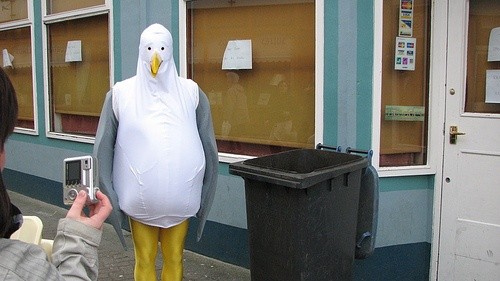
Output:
[62,155,101,205]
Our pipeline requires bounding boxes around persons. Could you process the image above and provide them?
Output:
[0,67,113,281]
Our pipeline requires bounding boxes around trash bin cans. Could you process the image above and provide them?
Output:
[229,143,378,281]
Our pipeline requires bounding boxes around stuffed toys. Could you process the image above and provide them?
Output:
[93,24,219,281]
